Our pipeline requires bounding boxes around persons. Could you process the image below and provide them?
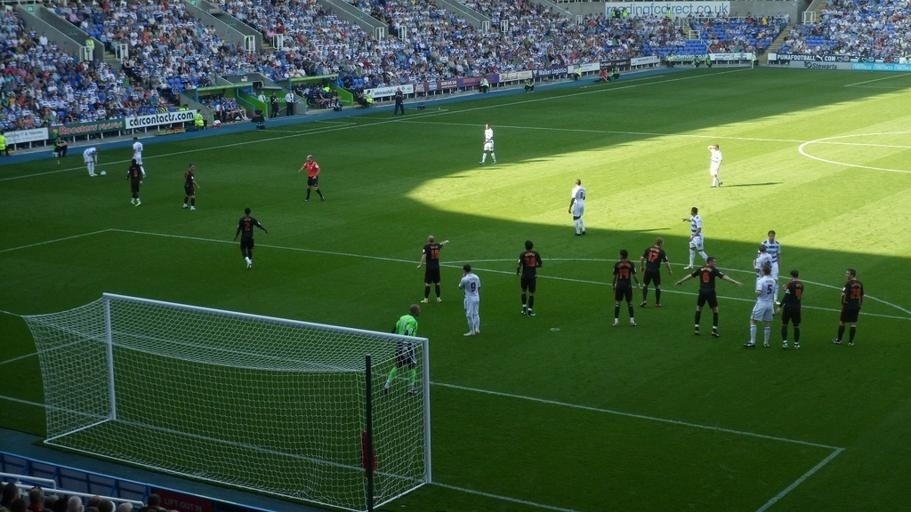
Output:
[777,0,911,65]
[1,132,10,157]
[383,302,421,395]
[479,123,496,166]
[612,6,790,69]
[1,465,180,511]
[126,159,143,207]
[132,137,146,177]
[234,207,268,270]
[683,207,709,270]
[832,268,864,346]
[639,237,673,308]
[183,164,200,211]
[569,179,587,237]
[708,143,723,189]
[417,233,449,304]
[743,231,805,351]
[607,248,641,327]
[54,136,68,159]
[299,154,325,202]
[332,0,637,116]
[674,255,743,337]
[459,264,481,337]
[1,1,344,140]
[83,146,100,176]
[516,240,542,318]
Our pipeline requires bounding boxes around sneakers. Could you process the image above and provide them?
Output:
[87,172,326,271]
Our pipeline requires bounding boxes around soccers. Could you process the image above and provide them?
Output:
[101,171,106,176]
